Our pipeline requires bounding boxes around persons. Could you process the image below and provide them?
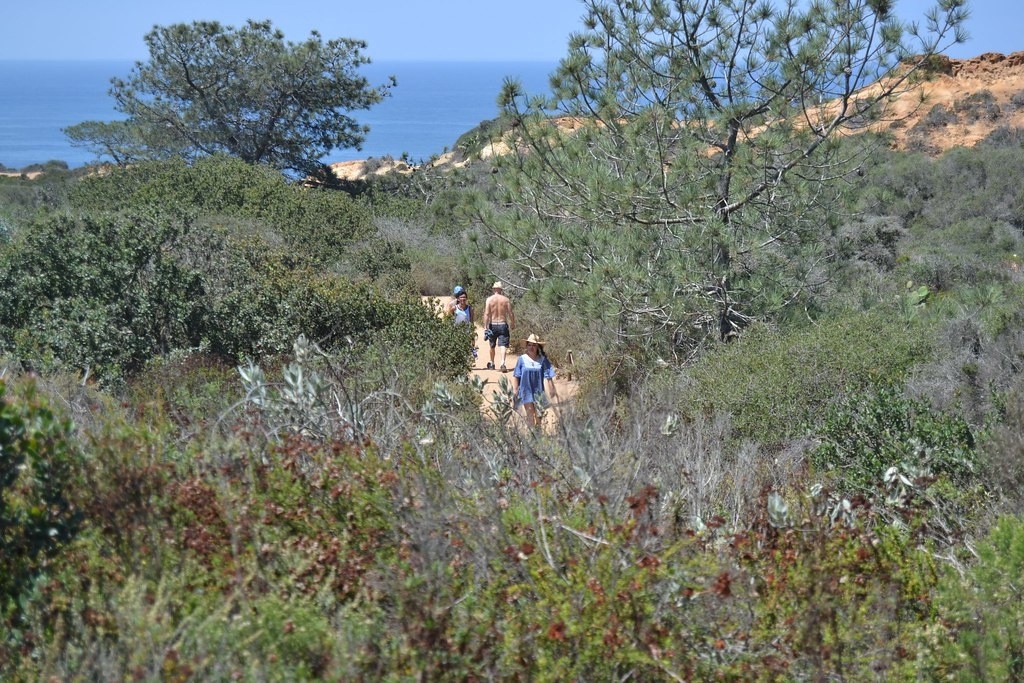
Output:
[513,334,556,431]
[484,282,515,372]
[447,286,476,367]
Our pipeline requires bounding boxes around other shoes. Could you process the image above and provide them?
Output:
[501,365,507,373]
[487,363,495,370]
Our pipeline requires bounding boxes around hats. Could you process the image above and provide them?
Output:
[492,281,503,289]
[453,285,463,294]
[525,333,545,344]
